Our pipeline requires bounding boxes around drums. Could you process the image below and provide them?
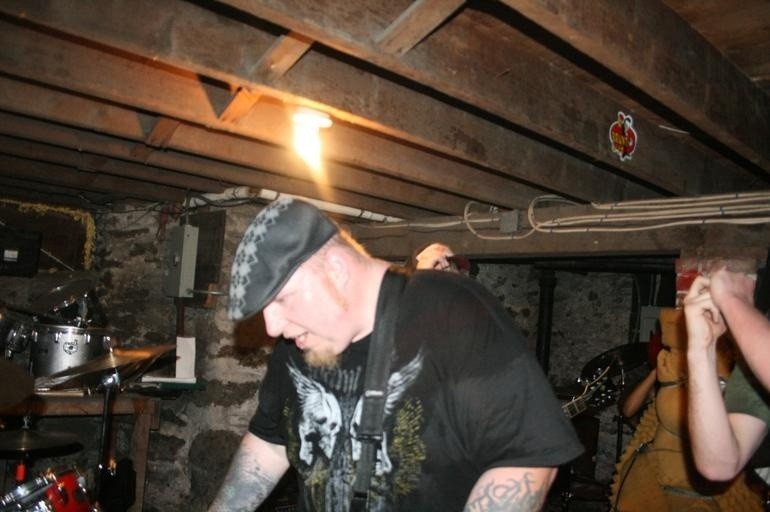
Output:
[0,300,107,387]
[0,463,95,510]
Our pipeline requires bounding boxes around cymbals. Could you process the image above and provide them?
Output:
[0,430,78,454]
[27,269,98,317]
[581,342,647,379]
[48,344,176,380]
[549,474,610,500]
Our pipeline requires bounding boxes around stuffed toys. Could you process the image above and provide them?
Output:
[609,302,766,511]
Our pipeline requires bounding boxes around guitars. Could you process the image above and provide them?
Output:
[560,367,617,419]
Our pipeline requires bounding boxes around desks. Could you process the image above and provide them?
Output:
[1,387,162,511]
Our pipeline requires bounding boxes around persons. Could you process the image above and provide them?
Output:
[616,330,664,429]
[404,242,471,278]
[204,194,589,512]
[681,266,770,485]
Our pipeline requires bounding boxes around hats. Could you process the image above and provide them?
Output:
[404,238,481,277]
[223,193,340,324]
